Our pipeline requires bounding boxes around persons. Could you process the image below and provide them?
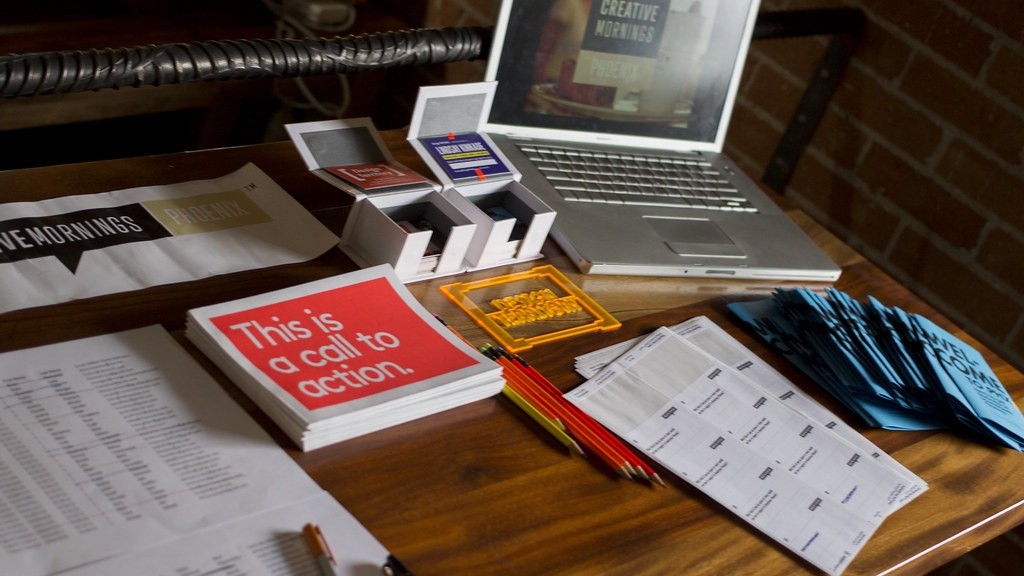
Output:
[535,0,593,85]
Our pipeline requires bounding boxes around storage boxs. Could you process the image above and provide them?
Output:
[283,82,558,285]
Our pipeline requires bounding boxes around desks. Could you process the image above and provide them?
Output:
[0,130,1024,576]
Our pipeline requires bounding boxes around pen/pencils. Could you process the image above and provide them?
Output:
[304,522,341,575]
[383,554,410,576]
[432,312,668,490]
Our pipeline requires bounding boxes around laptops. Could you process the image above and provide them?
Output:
[481,0,841,282]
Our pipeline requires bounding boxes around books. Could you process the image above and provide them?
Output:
[187,262,506,454]
